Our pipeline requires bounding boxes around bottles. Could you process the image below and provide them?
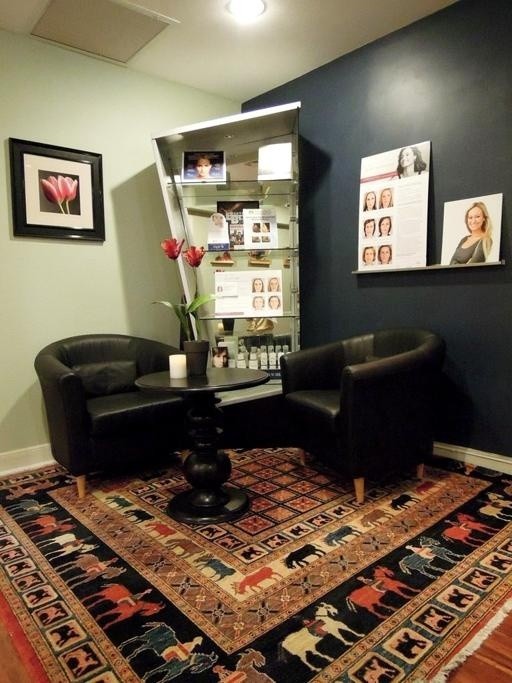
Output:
[236,344,291,370]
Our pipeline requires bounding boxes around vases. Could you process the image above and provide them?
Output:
[181,338,209,376]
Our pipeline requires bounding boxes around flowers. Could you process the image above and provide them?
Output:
[149,235,220,341]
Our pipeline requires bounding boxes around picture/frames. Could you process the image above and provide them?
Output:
[7,135,109,243]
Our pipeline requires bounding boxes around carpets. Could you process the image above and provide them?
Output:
[0,446,512,682]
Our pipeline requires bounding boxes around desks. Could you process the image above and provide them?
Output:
[133,366,272,524]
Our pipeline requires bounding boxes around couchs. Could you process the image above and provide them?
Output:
[34,331,186,500]
[279,325,447,504]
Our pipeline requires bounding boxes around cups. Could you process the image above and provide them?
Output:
[169,378,188,388]
[169,354,187,378]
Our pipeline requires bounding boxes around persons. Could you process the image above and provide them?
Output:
[252,295,266,310]
[389,145,429,180]
[212,346,228,367]
[267,276,281,291]
[212,211,271,246]
[378,215,391,237]
[362,246,376,266]
[362,218,375,237]
[379,186,393,208]
[377,244,392,264]
[191,152,213,179]
[250,277,264,292]
[448,201,495,264]
[267,295,281,310]
[364,191,376,211]
